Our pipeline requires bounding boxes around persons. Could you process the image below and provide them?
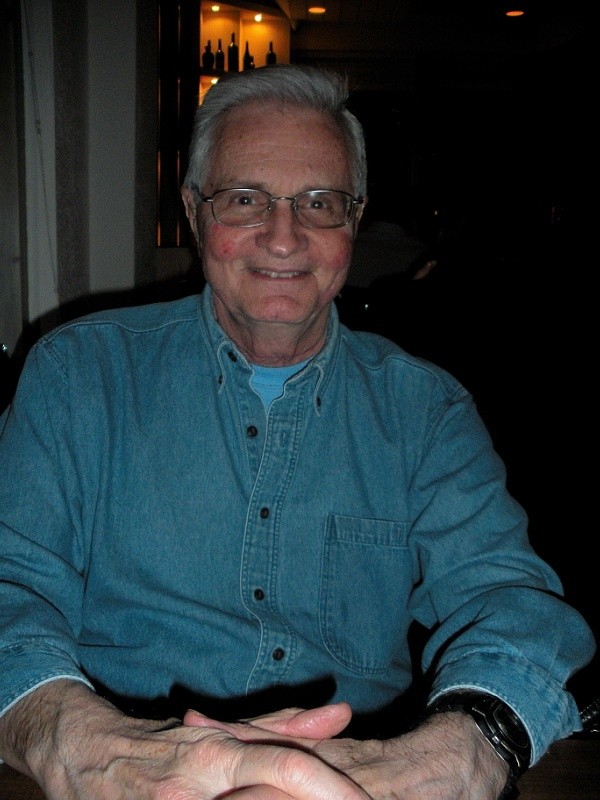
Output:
[3,69,594,799]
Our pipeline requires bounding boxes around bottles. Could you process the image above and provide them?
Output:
[202,31,278,75]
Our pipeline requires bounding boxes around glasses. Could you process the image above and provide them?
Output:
[191,180,363,228]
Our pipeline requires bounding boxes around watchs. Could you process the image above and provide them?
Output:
[417,688,531,798]
